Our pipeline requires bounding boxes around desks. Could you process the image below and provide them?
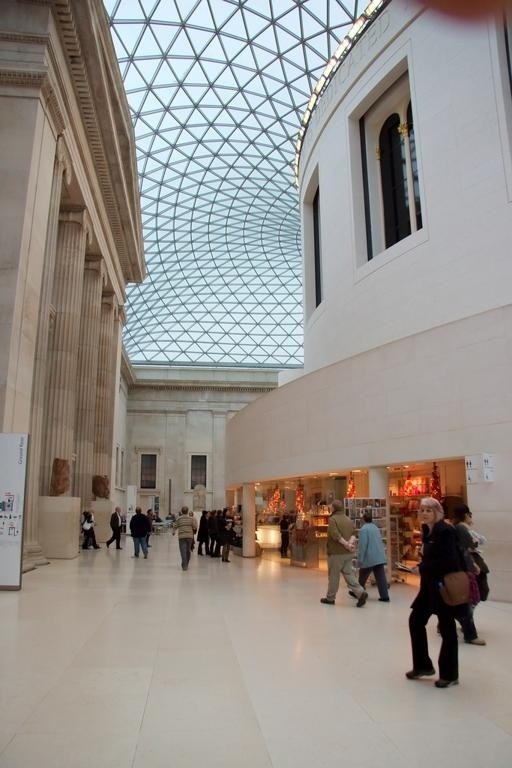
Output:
[152,518,174,535]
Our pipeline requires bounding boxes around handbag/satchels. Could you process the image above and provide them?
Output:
[440,571,471,606]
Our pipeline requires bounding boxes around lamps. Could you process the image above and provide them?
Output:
[289,0,387,190]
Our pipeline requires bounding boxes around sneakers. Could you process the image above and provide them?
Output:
[406,670,435,679]
[465,637,487,646]
[435,677,459,689]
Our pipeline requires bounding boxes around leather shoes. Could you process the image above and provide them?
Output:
[320,591,389,607]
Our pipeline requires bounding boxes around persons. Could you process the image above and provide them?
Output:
[348,512,390,602]
[436,505,486,647]
[81,504,292,571]
[320,499,367,608]
[404,495,468,688]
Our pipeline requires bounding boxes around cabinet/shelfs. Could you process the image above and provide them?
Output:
[342,495,391,585]
[312,510,331,560]
[389,500,404,570]
